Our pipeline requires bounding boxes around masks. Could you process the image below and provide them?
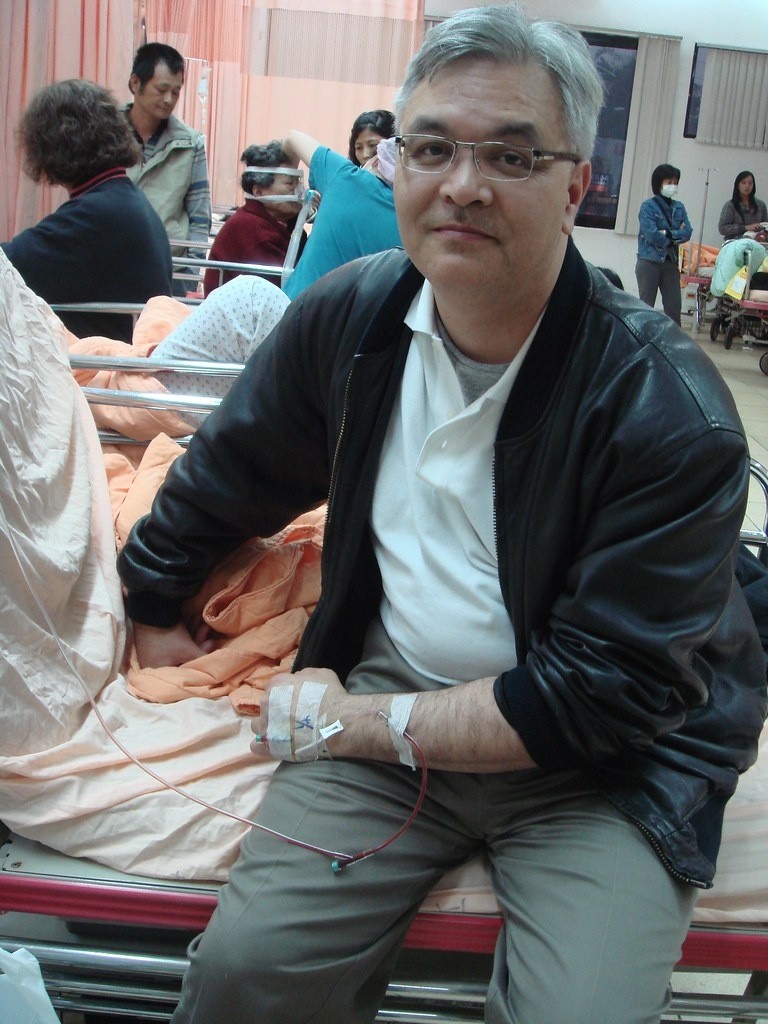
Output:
[660,185,678,198]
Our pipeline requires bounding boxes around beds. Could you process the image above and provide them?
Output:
[0,385,768,1024]
[710,239,768,350]
[36,202,314,446]
[677,236,723,334]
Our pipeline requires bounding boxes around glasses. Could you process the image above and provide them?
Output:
[395,134,581,182]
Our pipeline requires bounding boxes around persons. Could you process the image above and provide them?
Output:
[203,140,307,296]
[118,42,211,294]
[120,7,768,1024]
[636,164,693,325]
[283,133,404,303]
[719,171,768,327]
[2,79,173,341]
[349,110,396,167]
[65,276,291,437]
